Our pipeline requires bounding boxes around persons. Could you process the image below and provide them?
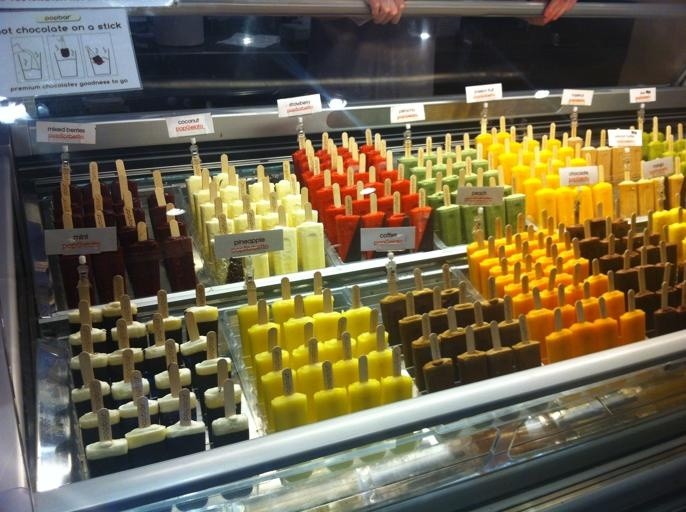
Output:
[365,0,578,27]
